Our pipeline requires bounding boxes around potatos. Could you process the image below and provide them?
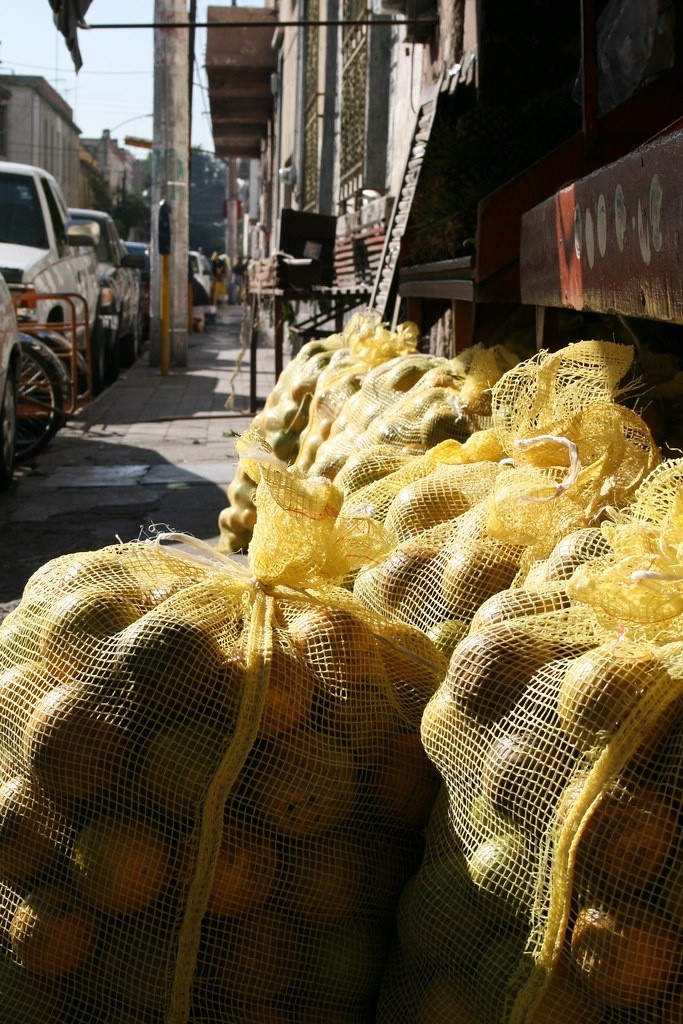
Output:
[0,342,683,1024]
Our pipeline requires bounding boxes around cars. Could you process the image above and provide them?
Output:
[0,161,216,496]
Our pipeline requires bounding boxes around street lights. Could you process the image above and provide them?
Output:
[89,112,154,162]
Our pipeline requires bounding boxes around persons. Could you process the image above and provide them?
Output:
[231,255,250,306]
[212,251,226,311]
